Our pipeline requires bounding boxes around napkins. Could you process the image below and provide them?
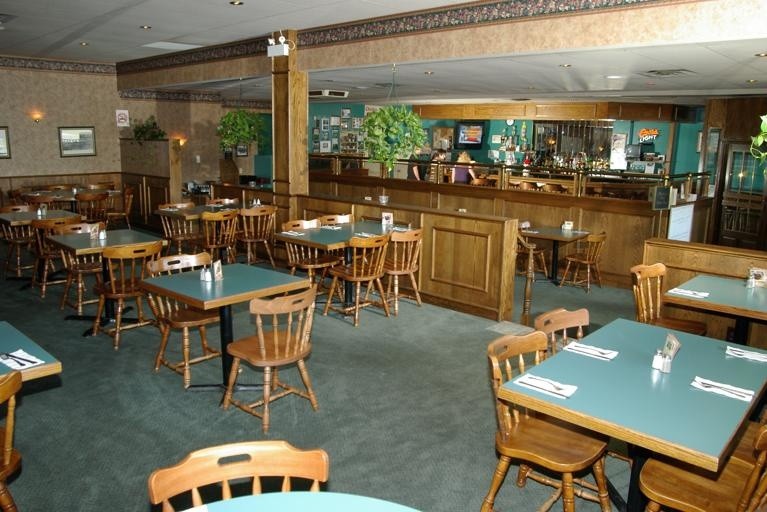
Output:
[2,347,45,372]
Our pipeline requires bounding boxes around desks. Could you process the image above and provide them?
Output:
[1,321,61,383]
[174,491,423,512]
[522,227,589,287]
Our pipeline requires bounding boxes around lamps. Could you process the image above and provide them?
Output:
[26,110,40,123]
[265,36,290,58]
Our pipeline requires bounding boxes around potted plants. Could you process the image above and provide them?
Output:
[355,106,426,180]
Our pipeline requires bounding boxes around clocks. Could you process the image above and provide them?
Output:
[504,119,516,127]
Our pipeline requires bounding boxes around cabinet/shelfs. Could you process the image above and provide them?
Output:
[337,129,362,155]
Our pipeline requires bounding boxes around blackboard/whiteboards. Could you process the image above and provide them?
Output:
[652,186,672,211]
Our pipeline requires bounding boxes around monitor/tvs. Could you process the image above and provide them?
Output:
[626,144,641,158]
[454,122,484,150]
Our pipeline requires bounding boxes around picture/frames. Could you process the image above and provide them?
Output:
[236,144,249,158]
[311,107,353,155]
[56,124,98,160]
[0,124,12,160]
[430,126,454,152]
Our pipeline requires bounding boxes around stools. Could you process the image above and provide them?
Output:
[471,178,494,187]
[517,181,648,200]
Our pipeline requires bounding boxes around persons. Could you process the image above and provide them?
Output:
[451,151,476,184]
[407,146,423,181]
[425,149,447,183]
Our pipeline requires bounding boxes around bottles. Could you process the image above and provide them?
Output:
[204,269,211,281]
[98,228,106,239]
[744,275,755,290]
[661,354,670,373]
[199,269,204,280]
[42,205,45,215]
[650,369,669,388]
[651,349,662,369]
[200,282,212,291]
[36,207,42,215]
[37,215,41,221]
[247,197,260,205]
[99,240,105,247]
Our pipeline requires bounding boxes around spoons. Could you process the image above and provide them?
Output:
[0,353,25,368]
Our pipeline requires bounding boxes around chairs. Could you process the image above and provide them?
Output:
[0,181,421,351]
[148,440,329,511]
[516,231,548,282]
[223,284,320,435]
[146,253,221,390]
[558,231,608,292]
[480,263,766,511]
[1,369,24,510]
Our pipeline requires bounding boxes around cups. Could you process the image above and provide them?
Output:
[249,181,256,187]
[379,195,387,204]
